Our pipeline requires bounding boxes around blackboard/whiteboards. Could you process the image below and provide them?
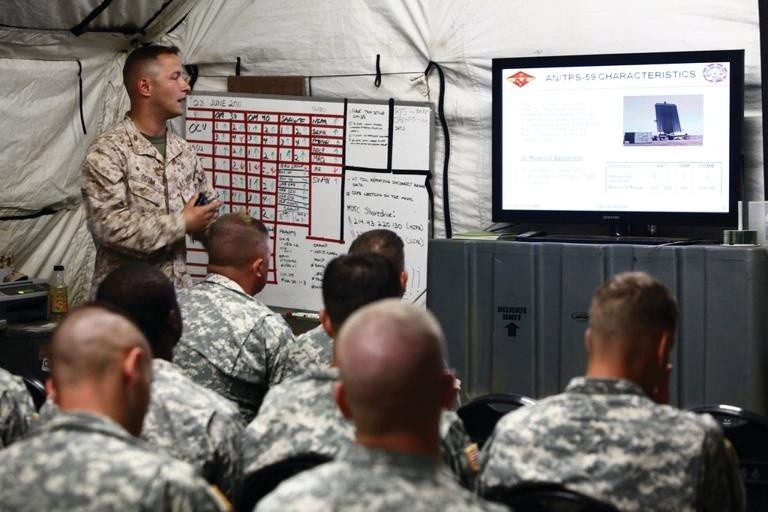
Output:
[178,89,435,318]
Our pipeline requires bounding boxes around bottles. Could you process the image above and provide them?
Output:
[0,255,16,283]
[47,265,70,322]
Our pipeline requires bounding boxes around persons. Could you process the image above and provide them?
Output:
[0,305,236,512]
[237,252,483,477]
[0,366,36,450]
[252,297,511,512]
[171,211,295,424]
[266,229,461,411]
[29,262,246,505]
[477,271,745,512]
[83,45,224,305]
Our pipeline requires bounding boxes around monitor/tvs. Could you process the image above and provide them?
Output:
[492,47,746,244]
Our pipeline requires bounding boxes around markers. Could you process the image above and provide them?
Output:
[289,311,320,320]
